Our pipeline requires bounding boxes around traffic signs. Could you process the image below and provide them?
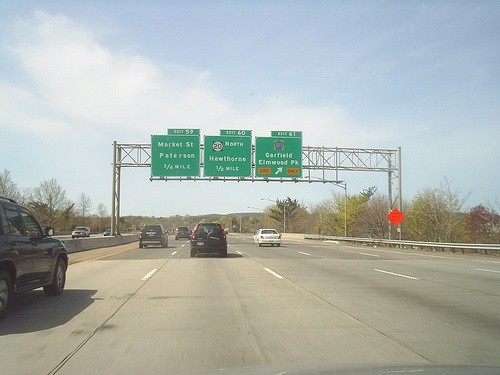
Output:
[255,135,302,178]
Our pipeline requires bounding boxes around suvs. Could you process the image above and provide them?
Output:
[175,226,191,240]
[71,226,90,238]
[139,224,169,248]
[0,195,69,322]
[189,222,227,257]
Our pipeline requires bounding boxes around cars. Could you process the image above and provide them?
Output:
[103,229,111,236]
[253,229,281,247]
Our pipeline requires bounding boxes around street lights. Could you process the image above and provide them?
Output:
[261,198,286,233]
[304,175,347,236]
